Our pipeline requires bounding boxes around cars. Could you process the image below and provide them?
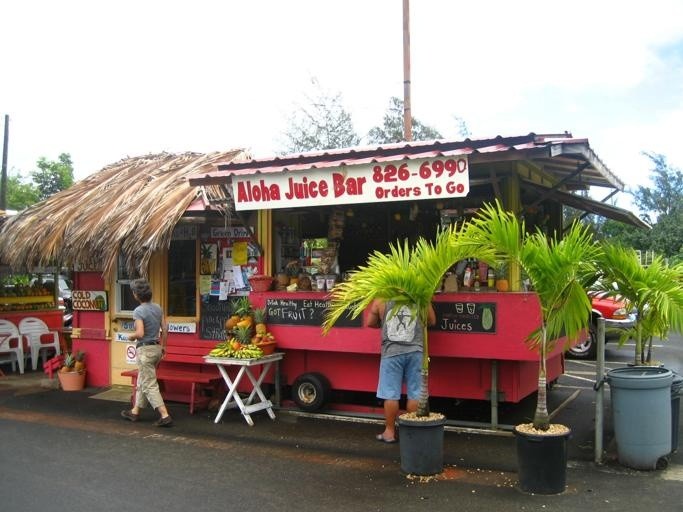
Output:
[565,281,650,359]
[5,273,72,327]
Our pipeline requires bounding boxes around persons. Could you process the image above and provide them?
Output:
[367,289,436,443]
[119,278,174,427]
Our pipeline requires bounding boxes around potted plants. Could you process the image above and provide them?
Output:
[321,219,507,477]
[580,239,683,459]
[453,197,607,496]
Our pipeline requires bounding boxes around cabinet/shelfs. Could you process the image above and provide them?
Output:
[277,243,300,274]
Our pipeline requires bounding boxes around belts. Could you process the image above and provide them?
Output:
[135,341,159,349]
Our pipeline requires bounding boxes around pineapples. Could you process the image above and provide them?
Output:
[253,307,267,334]
[225,296,252,320]
[61,352,76,371]
[201,244,215,274]
[74,350,86,370]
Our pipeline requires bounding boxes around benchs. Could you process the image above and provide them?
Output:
[120,338,230,415]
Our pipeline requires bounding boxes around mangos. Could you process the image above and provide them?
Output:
[237,320,251,328]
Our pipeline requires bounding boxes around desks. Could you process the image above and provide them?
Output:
[201,352,285,427]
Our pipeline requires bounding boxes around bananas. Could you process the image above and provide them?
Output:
[208,338,263,360]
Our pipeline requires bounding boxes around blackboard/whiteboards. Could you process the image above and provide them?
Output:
[260,298,365,328]
[435,302,494,334]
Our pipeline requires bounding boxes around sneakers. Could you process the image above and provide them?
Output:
[120,409,140,422]
[151,414,172,427]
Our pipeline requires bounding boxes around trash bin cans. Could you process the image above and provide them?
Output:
[609,367,674,469]
[198,293,248,342]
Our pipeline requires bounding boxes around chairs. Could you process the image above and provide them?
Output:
[18,317,61,371]
[0,319,25,375]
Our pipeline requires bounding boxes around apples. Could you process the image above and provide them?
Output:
[250,336,272,344]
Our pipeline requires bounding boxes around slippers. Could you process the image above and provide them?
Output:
[375,431,397,443]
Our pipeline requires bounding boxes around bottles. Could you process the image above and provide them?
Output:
[445,261,496,293]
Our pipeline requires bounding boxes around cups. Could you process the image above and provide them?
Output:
[317,278,335,290]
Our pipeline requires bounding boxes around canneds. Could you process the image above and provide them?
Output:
[472,276,481,291]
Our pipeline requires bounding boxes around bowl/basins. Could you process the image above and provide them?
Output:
[57,369,87,391]
[247,275,273,292]
[258,340,276,355]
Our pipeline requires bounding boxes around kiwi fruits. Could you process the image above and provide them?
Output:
[225,319,239,329]
